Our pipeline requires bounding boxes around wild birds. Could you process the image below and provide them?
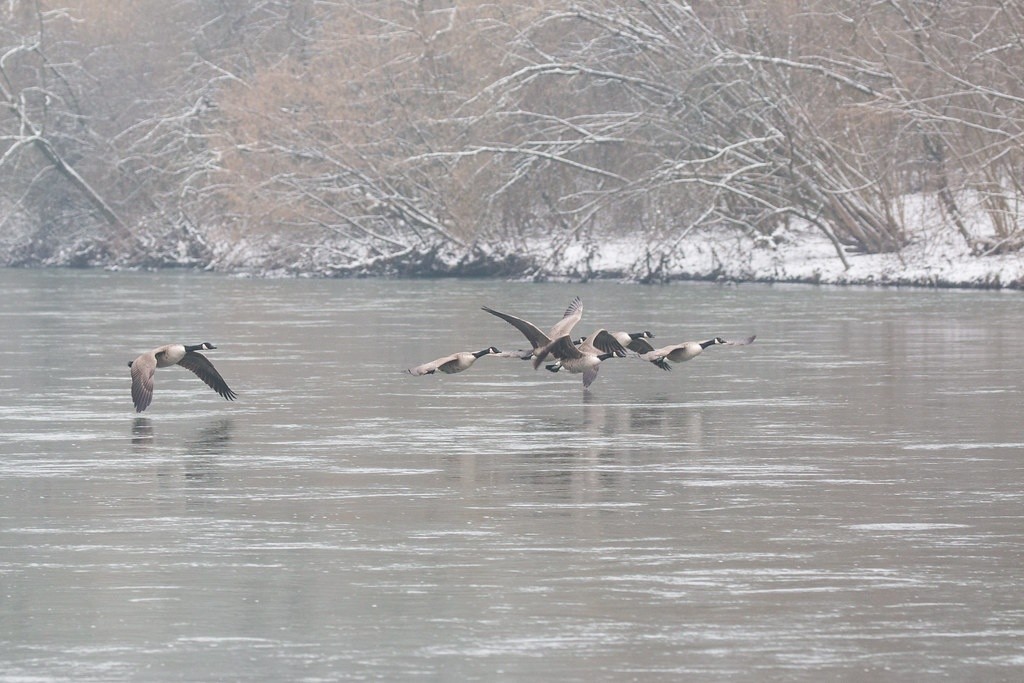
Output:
[481,297,757,389]
[400,346,529,376]
[127,342,239,413]
